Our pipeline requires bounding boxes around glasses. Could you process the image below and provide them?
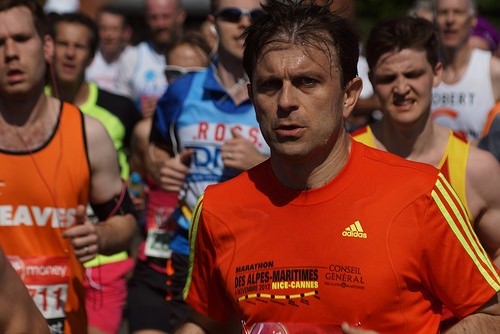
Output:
[214,6,264,24]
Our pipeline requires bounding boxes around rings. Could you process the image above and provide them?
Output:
[227,152,232,159]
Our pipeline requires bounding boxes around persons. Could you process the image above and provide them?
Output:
[0,0,500,334]
[171,0,500,334]
